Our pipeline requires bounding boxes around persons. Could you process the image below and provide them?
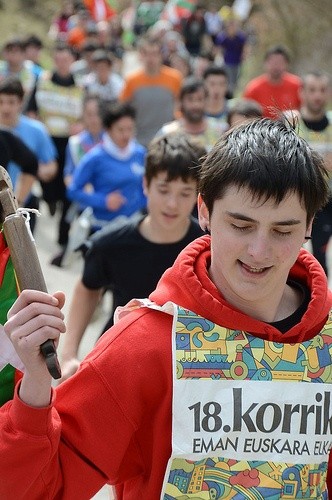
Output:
[0,104,332,500]
[228,98,266,128]
[25,40,84,265]
[149,76,222,164]
[0,127,39,222]
[69,105,152,324]
[0,0,305,142]
[63,97,112,218]
[0,78,57,239]
[281,71,332,280]
[51,130,211,393]
